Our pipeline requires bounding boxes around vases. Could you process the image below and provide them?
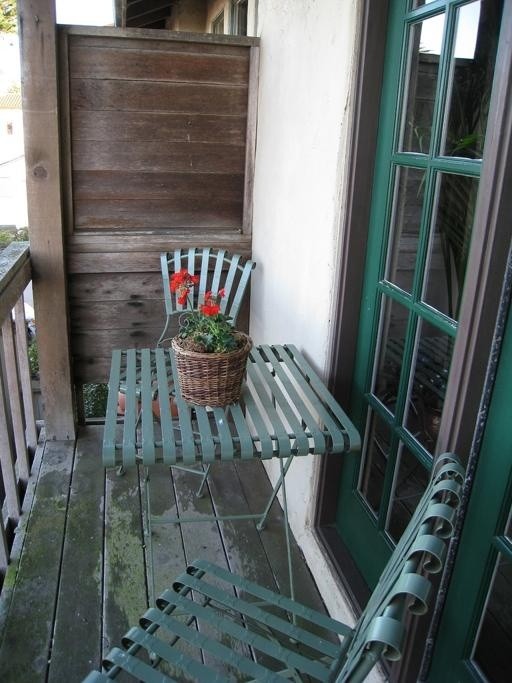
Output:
[172,332,253,406]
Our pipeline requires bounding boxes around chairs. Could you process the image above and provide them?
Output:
[107,246,256,499]
[81,453,466,683]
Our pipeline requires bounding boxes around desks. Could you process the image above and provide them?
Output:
[386,335,456,401]
[101,349,360,643]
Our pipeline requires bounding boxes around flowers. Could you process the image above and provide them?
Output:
[170,270,239,354]
